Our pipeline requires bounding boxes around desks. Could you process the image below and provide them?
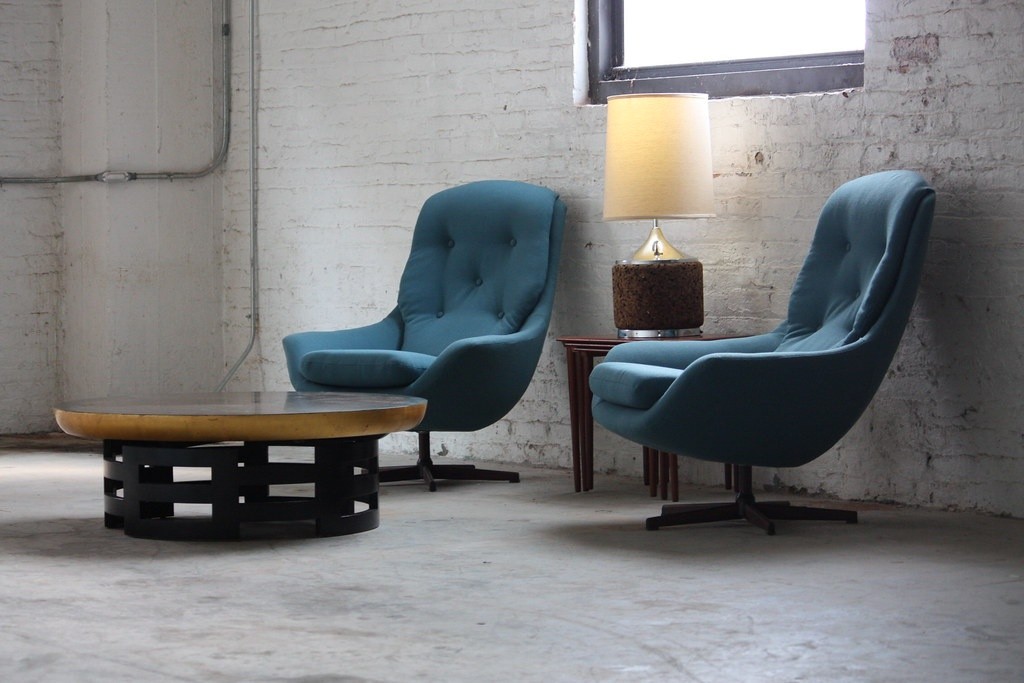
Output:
[556,333,756,503]
[52,391,427,544]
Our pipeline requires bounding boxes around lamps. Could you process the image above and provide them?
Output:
[601,92,719,338]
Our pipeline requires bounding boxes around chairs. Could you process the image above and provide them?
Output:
[281,179,567,492]
[588,171,937,535]
